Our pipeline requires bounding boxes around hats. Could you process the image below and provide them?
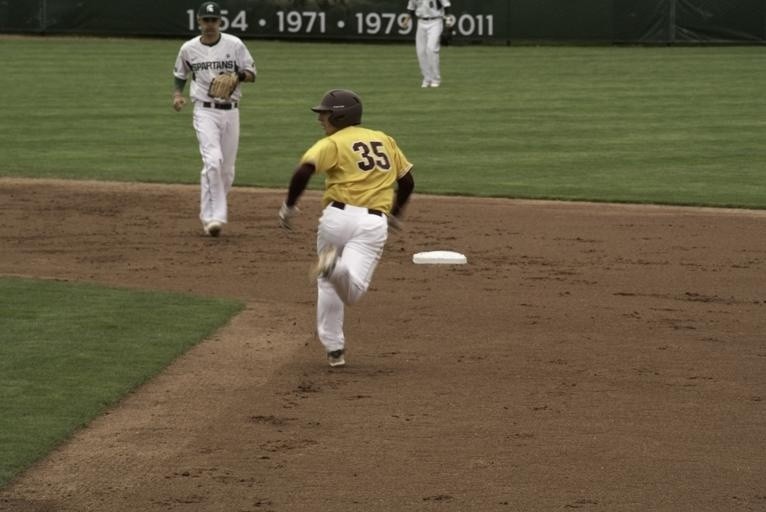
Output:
[198,1,221,20]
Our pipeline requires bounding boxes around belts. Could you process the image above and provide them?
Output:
[203,102,238,110]
[419,15,443,20]
[329,200,385,217]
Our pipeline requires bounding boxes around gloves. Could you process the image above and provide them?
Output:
[278,201,302,233]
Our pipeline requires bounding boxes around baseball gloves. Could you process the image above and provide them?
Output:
[207,70,240,100]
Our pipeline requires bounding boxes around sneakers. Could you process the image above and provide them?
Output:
[326,348,345,367]
[204,221,222,237]
[421,79,441,89]
[309,243,338,284]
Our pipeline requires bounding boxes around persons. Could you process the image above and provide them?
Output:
[405,0,453,89]
[170,0,258,238]
[277,89,417,368]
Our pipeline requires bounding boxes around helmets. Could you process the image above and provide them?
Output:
[311,89,362,128]
